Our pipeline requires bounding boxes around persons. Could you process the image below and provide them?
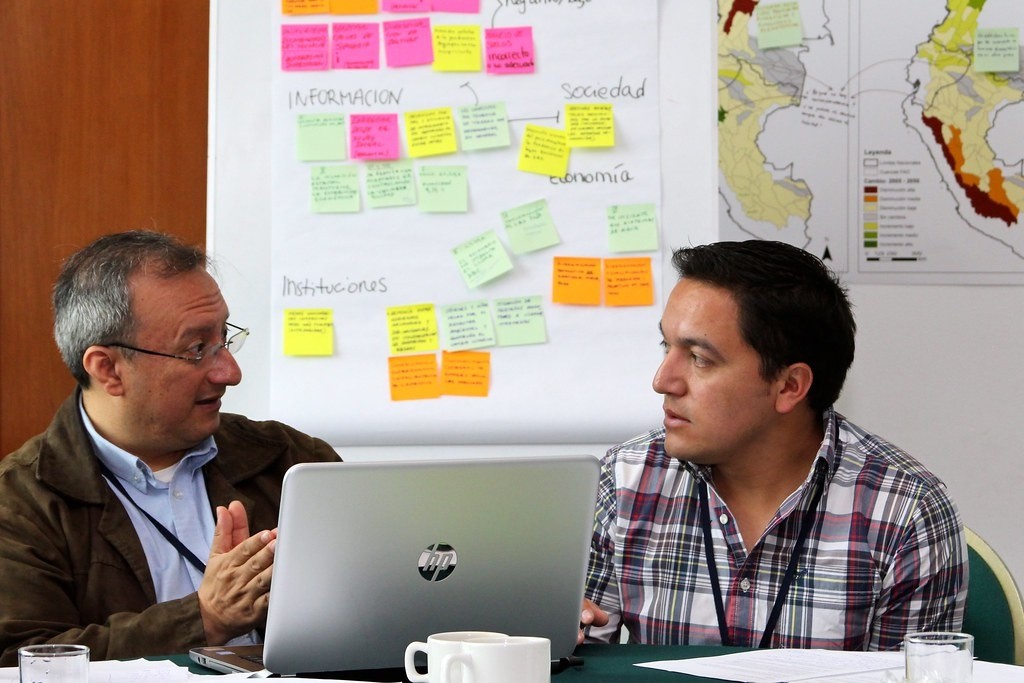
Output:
[0,228,345,669]
[576,240,970,653]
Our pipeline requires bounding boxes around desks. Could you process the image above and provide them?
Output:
[137,642,774,683]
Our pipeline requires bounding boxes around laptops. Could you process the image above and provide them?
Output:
[191,457,601,679]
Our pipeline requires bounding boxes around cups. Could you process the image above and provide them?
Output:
[18,643,90,683]
[404,632,509,683]
[440,636,551,683]
[904,631,975,683]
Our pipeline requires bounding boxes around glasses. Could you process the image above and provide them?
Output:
[101,321,251,371]
